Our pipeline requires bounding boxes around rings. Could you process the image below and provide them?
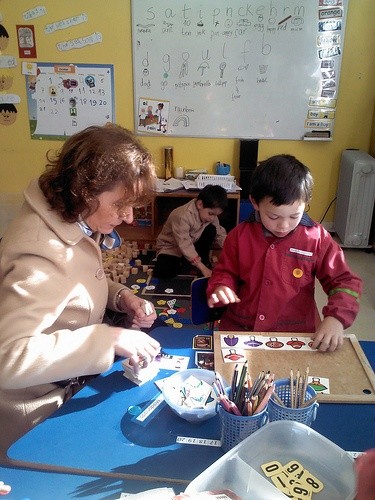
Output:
[140,354,149,361]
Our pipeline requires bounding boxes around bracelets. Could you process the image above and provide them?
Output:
[116,290,124,312]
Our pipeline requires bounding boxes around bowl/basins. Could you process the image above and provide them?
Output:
[164,369,227,423]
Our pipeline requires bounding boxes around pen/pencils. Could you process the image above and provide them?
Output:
[211,361,324,417]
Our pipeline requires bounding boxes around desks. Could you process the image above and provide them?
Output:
[148,177,240,238]
[0,327,375,500]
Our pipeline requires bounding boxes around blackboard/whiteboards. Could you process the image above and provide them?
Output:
[130,0,349,141]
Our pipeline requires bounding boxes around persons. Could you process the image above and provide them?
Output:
[0,122,162,464]
[205,154,362,352]
[153,184,228,279]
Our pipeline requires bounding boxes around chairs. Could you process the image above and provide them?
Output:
[191,275,222,330]
